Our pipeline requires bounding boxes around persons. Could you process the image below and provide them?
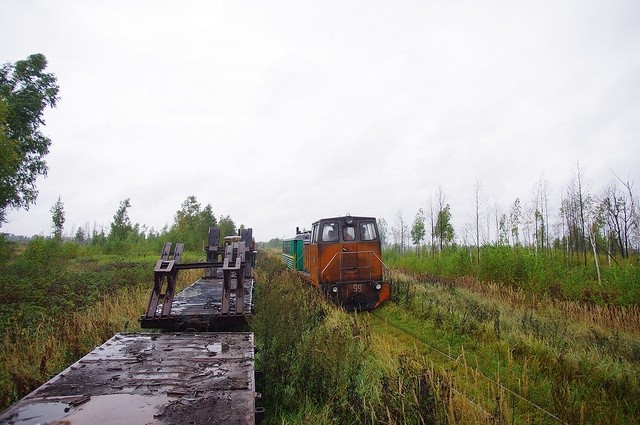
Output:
[344,228,353,240]
[328,230,334,239]
[360,227,366,240]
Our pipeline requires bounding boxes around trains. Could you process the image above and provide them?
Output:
[282,212,391,312]
